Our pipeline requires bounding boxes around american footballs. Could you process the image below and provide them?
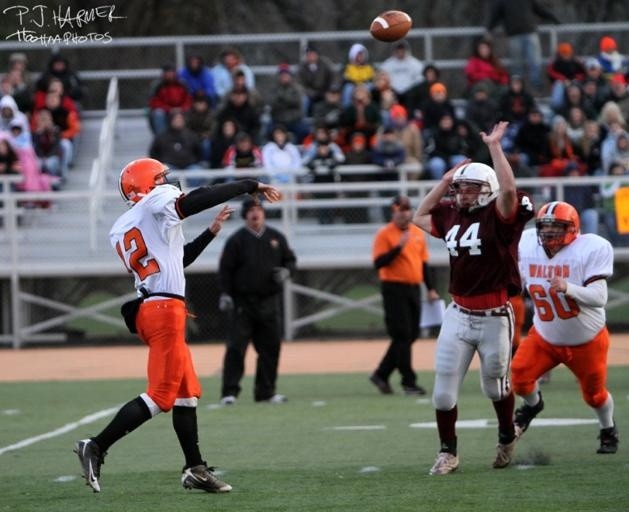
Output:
[371,11,412,40]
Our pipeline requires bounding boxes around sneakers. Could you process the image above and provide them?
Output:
[221,394,287,403]
[596,419,620,454]
[429,451,460,475]
[369,375,427,396]
[493,435,518,468]
[74,438,105,493]
[512,391,544,439]
[181,463,232,494]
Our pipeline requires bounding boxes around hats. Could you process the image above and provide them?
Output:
[391,197,411,212]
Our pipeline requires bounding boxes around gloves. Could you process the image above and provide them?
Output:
[219,293,234,311]
[273,266,291,284]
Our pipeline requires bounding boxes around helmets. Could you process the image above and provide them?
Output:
[534,202,580,250]
[451,162,499,211]
[117,158,169,207]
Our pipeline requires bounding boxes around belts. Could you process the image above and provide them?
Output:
[453,304,509,319]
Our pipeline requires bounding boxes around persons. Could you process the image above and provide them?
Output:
[506,285,552,385]
[412,120,534,474]
[370,197,439,394]
[73,159,282,494]
[216,198,297,405]
[508,201,620,454]
[144,2,629,246]
[0,50,82,226]
